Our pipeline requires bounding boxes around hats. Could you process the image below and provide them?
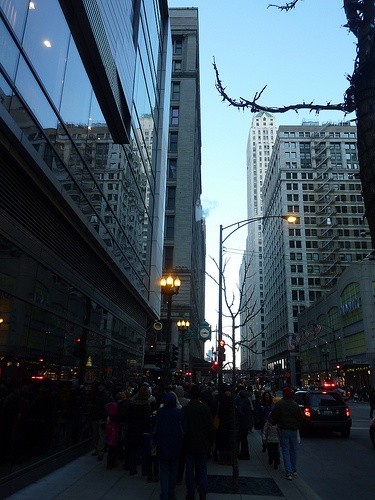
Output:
[283,388,291,396]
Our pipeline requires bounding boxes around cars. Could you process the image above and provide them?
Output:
[292,389,353,439]
[333,388,351,400]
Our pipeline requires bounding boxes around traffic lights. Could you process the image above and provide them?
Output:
[173,344,178,361]
[218,341,225,361]
[214,362,217,369]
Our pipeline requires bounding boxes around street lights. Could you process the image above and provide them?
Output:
[218,214,298,386]
[321,347,329,380]
[158,275,182,365]
[177,321,191,374]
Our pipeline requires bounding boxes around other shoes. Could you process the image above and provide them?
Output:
[293,472,297,476]
[147,476,152,481]
[154,476,159,482]
[288,475,292,480]
[97,456,103,460]
[130,471,137,475]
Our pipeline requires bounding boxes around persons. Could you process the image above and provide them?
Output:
[92,375,375,500]
